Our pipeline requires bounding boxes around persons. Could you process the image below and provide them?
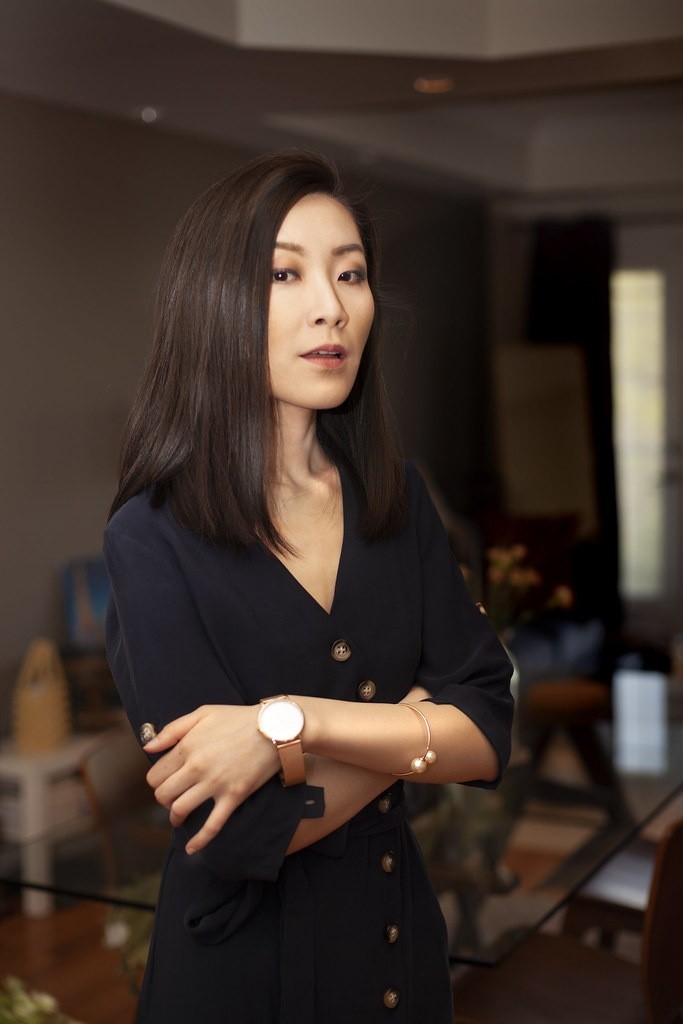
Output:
[101,149,514,1024]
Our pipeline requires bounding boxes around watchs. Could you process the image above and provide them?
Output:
[258,694,306,787]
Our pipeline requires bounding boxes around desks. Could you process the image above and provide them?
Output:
[0,673,682,969]
[0,736,115,918]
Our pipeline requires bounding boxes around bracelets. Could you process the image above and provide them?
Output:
[391,702,435,776]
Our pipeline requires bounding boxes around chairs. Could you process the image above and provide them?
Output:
[563,820,682,952]
[448,821,683,1024]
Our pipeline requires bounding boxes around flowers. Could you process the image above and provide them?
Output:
[462,543,571,609]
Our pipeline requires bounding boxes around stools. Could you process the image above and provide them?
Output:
[486,680,626,865]
